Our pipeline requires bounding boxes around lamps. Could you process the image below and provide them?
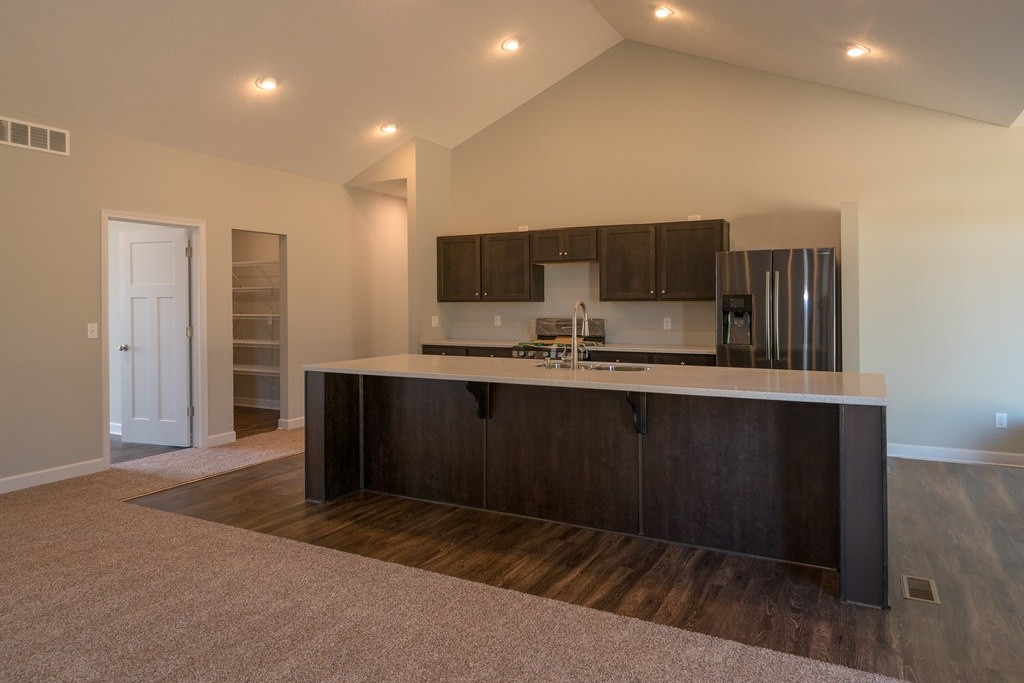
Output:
[501,38,522,52]
[650,6,674,18]
[845,44,871,57]
[380,122,397,134]
[255,75,280,90]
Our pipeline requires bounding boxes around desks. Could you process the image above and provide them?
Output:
[302,354,891,610]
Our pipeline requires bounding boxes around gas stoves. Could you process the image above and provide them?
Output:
[513,341,598,359]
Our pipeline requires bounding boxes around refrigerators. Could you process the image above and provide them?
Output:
[715,247,841,371]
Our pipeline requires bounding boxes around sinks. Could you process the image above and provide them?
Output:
[589,363,652,372]
[533,361,590,370]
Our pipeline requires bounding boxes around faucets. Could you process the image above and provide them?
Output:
[570,300,589,370]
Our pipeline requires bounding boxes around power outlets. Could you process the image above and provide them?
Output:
[88,323,97,339]
[664,318,672,329]
[494,316,501,326]
[995,413,1007,428]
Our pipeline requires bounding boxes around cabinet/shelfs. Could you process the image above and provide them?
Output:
[437,231,545,302]
[599,219,730,302]
[232,261,280,411]
[530,226,598,264]
[422,347,717,367]
